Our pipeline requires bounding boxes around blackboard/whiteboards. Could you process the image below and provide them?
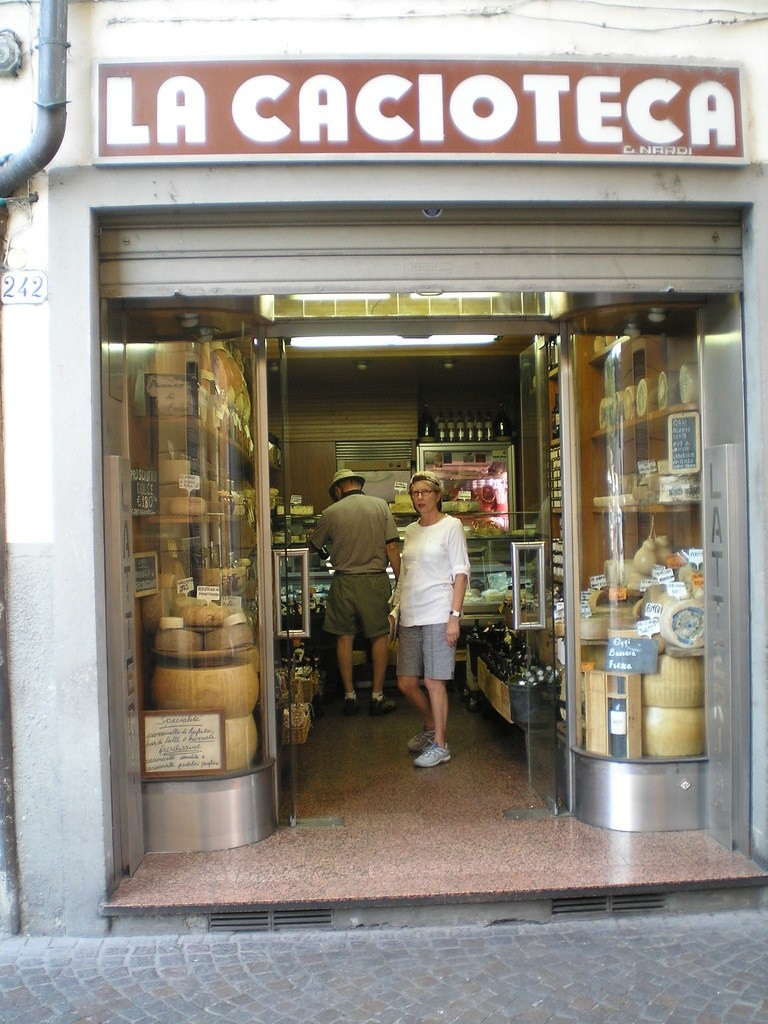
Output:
[605,637,659,672]
[132,551,160,598]
[182,536,203,566]
[668,412,701,474]
[130,468,161,516]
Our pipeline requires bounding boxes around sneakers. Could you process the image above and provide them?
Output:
[407,726,435,751]
[369,695,396,715]
[414,743,451,767]
[344,694,360,716]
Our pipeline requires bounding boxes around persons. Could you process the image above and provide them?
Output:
[397,471,470,768]
[309,469,400,715]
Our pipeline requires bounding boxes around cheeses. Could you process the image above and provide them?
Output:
[389,494,479,512]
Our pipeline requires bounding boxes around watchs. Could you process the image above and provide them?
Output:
[450,610,461,618]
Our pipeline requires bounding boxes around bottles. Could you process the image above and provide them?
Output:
[437,411,493,442]
[552,393,560,439]
[547,338,559,371]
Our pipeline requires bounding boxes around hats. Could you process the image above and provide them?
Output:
[409,470,443,514]
[328,468,365,500]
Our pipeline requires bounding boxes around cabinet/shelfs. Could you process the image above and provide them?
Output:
[537,329,702,695]
[137,370,283,651]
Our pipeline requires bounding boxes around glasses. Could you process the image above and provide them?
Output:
[408,489,437,497]
